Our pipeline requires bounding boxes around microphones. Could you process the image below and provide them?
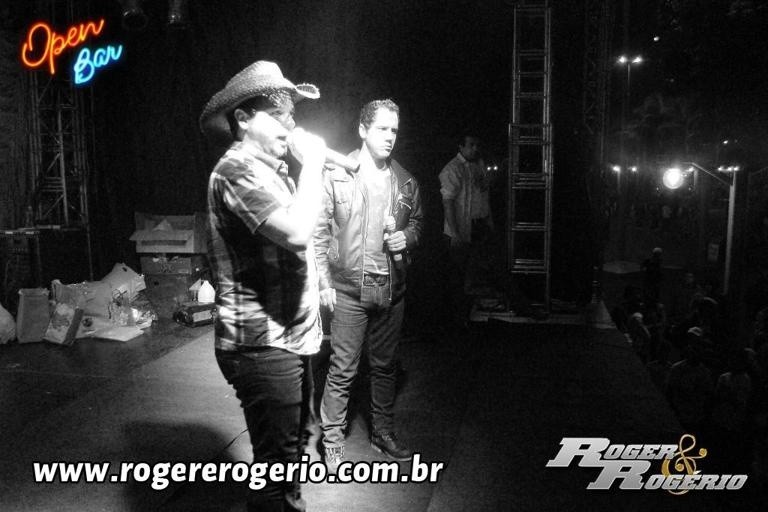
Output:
[285,131,360,173]
[384,216,403,270]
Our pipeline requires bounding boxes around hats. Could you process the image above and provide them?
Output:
[197,58,321,149]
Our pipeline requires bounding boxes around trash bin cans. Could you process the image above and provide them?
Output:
[16,288,49,344]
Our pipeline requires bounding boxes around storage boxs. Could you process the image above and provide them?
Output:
[128,211,209,255]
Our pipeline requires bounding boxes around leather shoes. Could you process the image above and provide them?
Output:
[324,446,343,475]
[370,431,412,461]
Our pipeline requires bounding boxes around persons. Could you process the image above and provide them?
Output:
[610,269,768,512]
[314,98,426,474]
[640,247,669,305]
[197,58,327,512]
[437,120,550,321]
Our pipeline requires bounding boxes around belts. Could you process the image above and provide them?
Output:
[363,273,390,287]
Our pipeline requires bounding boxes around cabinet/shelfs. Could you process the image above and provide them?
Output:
[139,254,203,320]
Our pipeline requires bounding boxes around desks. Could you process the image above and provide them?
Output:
[0,228,89,293]
